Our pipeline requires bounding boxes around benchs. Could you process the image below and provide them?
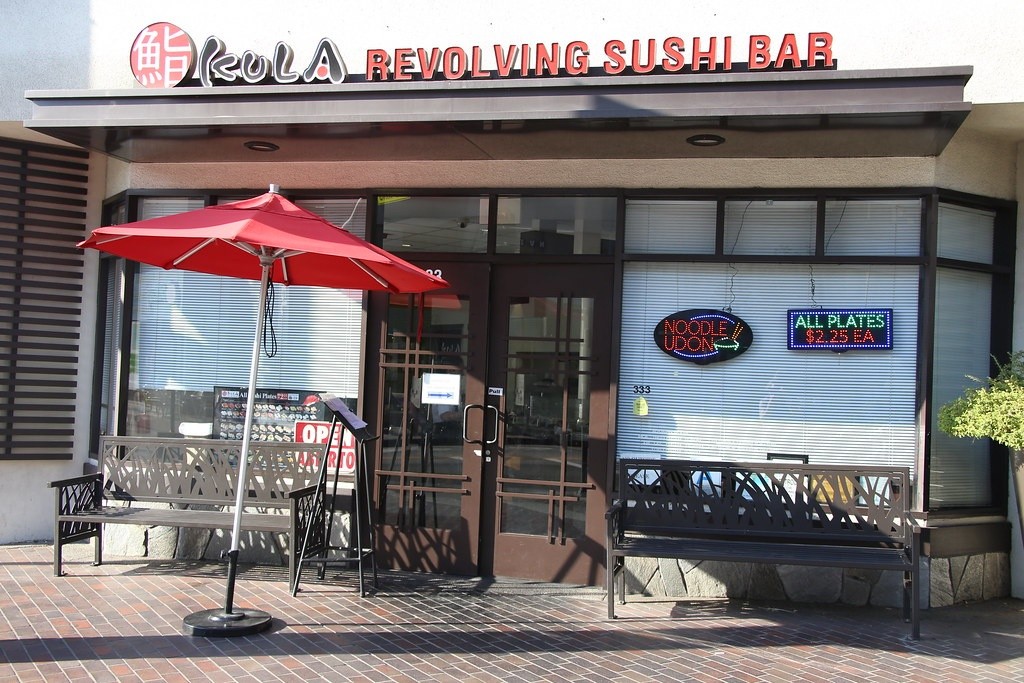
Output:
[45,436,329,592]
[604,457,922,642]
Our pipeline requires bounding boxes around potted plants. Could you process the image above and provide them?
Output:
[937,347,1023,551]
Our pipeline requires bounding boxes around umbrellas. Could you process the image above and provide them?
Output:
[74,184,455,638]
